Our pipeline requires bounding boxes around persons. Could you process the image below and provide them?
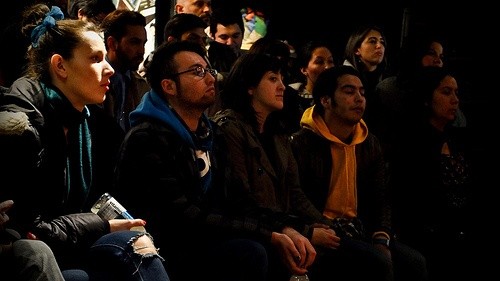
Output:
[0,4,169,281]
[0,199,65,281]
[67,0,500,281]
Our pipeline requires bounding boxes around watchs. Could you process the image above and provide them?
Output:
[372,238,390,247]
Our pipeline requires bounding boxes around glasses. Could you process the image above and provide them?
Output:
[178,66,218,77]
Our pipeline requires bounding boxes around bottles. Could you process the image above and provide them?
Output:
[91,192,146,233]
[289,265,309,281]
